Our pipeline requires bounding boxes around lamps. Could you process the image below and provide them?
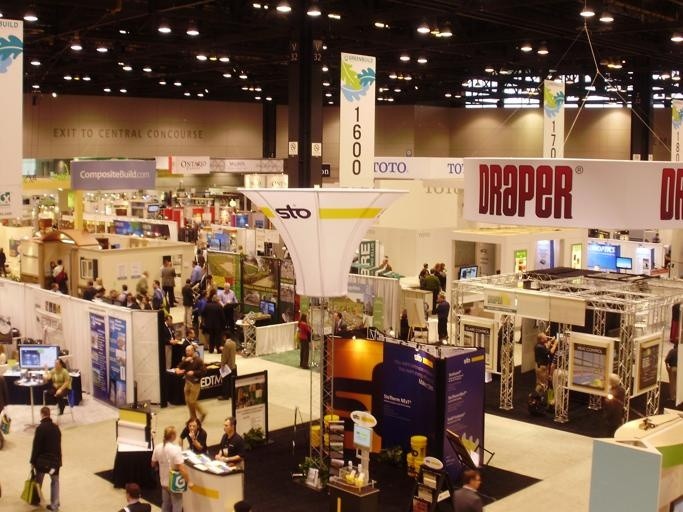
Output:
[417,21,430,34]
[276,1,292,12]
[599,11,615,22]
[671,31,682,41]
[537,46,549,54]
[440,24,452,37]
[307,5,321,16]
[521,42,533,52]
[579,6,595,17]
[23,10,38,21]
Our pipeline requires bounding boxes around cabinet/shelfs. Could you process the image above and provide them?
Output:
[406,457,452,511]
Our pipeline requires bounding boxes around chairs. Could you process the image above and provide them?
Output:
[40,375,75,427]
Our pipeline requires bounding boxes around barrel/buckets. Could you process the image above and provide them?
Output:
[406,453,419,477]
[410,435,428,462]
[323,433,330,452]
[311,425,321,449]
[413,457,425,480]
[323,415,340,436]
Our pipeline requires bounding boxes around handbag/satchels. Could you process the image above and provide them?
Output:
[169,471,188,494]
[37,453,58,472]
[21,469,40,505]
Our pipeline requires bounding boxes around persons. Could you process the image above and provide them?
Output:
[531,331,559,408]
[664,337,679,401]
[209,416,244,468]
[296,254,451,370]
[233,499,251,512]
[177,415,208,455]
[116,482,151,512]
[29,406,62,511]
[450,470,483,511]
[1,247,242,425]
[150,426,195,511]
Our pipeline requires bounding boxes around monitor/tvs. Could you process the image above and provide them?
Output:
[259,300,276,315]
[216,287,224,296]
[352,423,374,452]
[459,265,478,279]
[148,205,159,213]
[18,344,60,371]
[616,256,632,269]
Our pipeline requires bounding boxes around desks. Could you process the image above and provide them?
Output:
[163,360,236,403]
[14,377,48,434]
[0,369,82,404]
[235,318,255,357]
